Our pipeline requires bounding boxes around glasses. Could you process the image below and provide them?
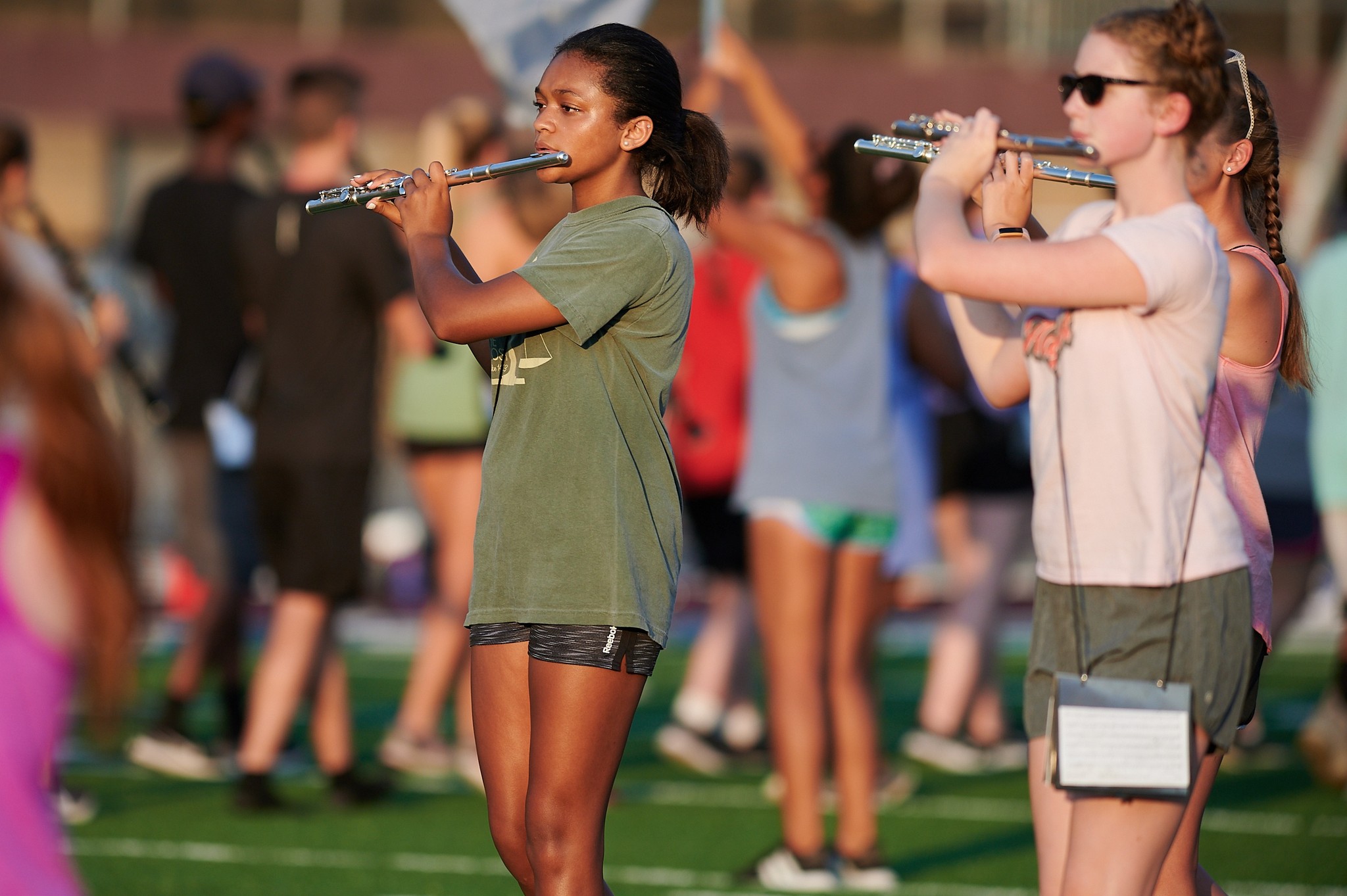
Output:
[1058,72,1161,107]
[1223,47,1254,139]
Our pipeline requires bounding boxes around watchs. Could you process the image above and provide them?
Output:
[989,226,1032,242]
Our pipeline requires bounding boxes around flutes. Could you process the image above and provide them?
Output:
[852,133,1119,190]
[304,150,570,216]
[889,113,1100,161]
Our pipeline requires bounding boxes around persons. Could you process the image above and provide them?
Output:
[0,116,128,830]
[342,26,733,896]
[113,53,1346,787]
[890,2,1256,895]
[0,254,130,894]
[970,69,1305,894]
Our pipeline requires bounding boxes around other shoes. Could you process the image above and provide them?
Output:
[651,708,769,779]
[232,774,312,814]
[57,788,98,826]
[1299,703,1347,780]
[377,735,488,794]
[902,725,1034,780]
[123,729,250,786]
[730,843,900,894]
[322,769,396,808]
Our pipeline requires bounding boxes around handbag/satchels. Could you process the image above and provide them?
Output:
[1041,670,1194,804]
